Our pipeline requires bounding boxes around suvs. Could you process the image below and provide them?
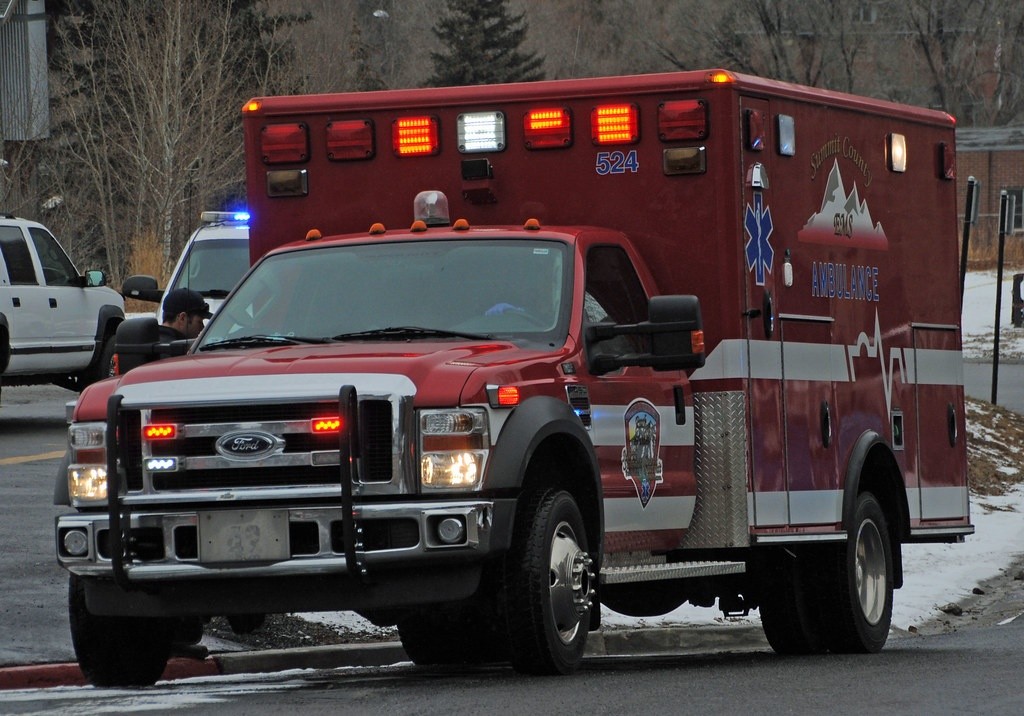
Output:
[0,214,127,395]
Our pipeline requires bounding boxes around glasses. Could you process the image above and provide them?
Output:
[178,303,209,317]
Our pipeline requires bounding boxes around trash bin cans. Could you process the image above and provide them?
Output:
[1011,273,1024,328]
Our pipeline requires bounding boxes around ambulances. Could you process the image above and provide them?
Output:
[55,67,976,689]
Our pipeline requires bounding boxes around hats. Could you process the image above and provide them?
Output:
[163,287,214,319]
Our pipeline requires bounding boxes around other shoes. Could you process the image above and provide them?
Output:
[171,644,209,658]
[231,614,261,636]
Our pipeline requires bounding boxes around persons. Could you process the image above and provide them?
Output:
[155,287,215,659]
[484,259,614,323]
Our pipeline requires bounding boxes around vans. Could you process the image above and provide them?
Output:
[122,210,254,330]
